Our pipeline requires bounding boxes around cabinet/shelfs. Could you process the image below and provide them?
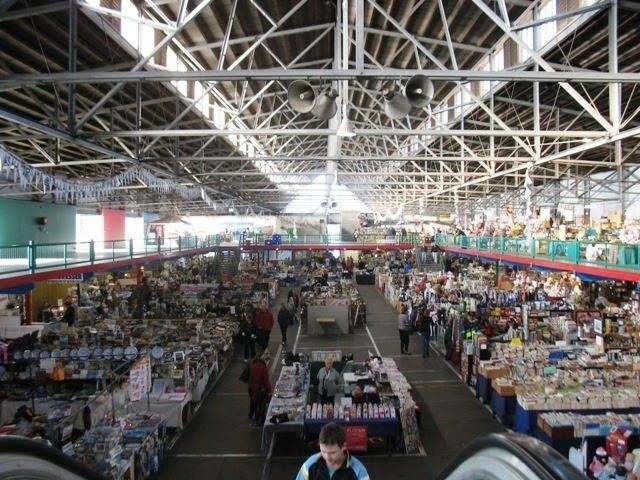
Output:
[279,271,375,335]
[259,350,424,458]
[2,272,279,480]
[375,272,639,478]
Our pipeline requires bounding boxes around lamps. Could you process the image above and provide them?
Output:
[336,105,356,137]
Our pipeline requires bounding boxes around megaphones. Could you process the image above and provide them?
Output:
[401,74,434,108]
[330,202,337,209]
[287,79,320,114]
[321,201,328,207]
[311,89,339,121]
[382,88,411,120]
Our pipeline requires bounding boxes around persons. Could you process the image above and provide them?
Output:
[246,351,273,421]
[251,382,270,428]
[60,300,76,327]
[315,358,345,407]
[240,286,300,363]
[30,426,54,448]
[351,382,369,407]
[278,253,366,286]
[419,309,438,357]
[295,419,371,480]
[398,306,414,354]
[380,223,492,251]
[443,309,522,362]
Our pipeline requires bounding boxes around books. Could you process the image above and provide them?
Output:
[480,341,640,438]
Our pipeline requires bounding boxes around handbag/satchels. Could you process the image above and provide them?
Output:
[416,321,424,332]
[238,366,250,383]
